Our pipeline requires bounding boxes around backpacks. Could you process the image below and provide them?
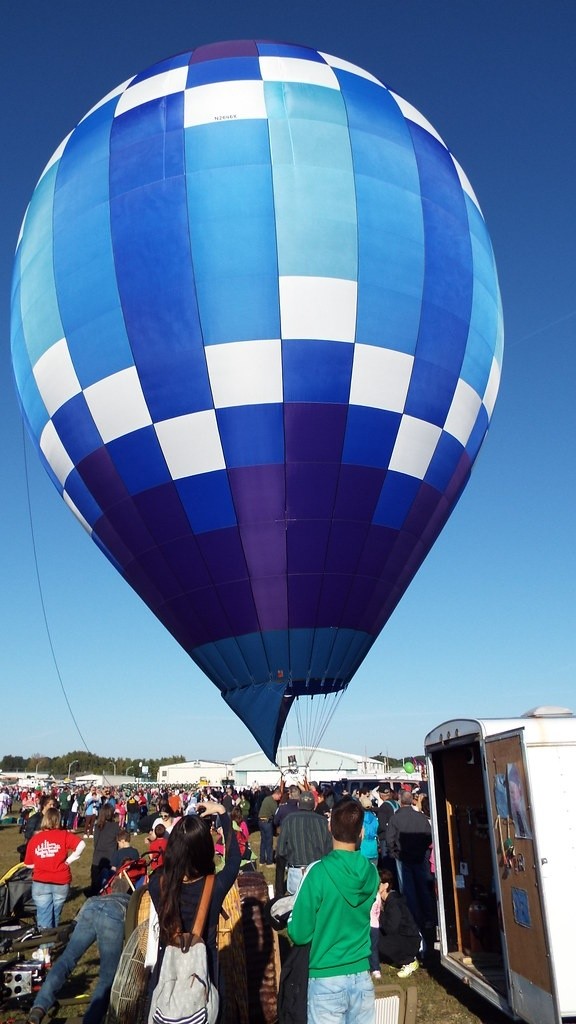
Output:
[148,873,219,1024]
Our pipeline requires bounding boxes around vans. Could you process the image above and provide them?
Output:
[347,779,422,793]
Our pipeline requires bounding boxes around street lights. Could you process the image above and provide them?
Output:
[125,765,133,776]
[67,760,79,779]
[36,761,43,774]
[109,761,117,776]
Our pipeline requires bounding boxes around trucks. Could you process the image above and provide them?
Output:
[423,704,576,1024]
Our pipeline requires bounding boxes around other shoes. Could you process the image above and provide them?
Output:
[372,970,382,979]
[25,1008,47,1024]
[259,863,276,868]
[83,834,94,839]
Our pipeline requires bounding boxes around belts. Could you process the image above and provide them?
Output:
[288,865,307,868]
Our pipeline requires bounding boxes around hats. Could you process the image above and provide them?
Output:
[308,781,317,787]
[361,797,372,808]
[376,785,390,793]
[298,791,316,811]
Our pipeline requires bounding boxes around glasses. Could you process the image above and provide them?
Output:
[159,813,169,818]
[309,783,314,786]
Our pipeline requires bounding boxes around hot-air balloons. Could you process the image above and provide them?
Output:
[6,36,510,793]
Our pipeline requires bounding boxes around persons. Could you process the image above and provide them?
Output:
[0,777,433,1024]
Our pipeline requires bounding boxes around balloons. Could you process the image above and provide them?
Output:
[404,762,414,773]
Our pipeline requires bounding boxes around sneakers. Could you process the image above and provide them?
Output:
[396,959,419,978]
[33,949,51,963]
